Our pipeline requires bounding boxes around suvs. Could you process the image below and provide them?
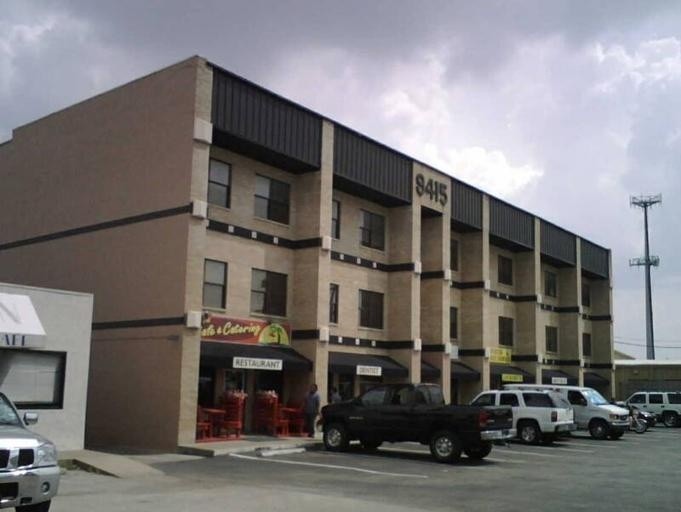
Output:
[615,390,681,428]
[0,391,60,512]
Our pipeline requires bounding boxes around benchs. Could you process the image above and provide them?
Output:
[196,405,211,442]
[215,397,243,438]
[254,398,288,436]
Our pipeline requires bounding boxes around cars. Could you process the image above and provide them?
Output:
[613,400,656,434]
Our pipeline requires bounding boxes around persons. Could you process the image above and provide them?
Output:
[301,383,321,438]
[330,385,342,404]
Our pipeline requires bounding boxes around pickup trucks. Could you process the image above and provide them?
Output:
[320,382,514,463]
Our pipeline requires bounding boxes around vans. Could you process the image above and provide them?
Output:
[469,389,578,445]
[504,383,631,441]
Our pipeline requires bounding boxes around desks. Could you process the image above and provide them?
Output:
[203,407,226,437]
[282,407,296,436]
